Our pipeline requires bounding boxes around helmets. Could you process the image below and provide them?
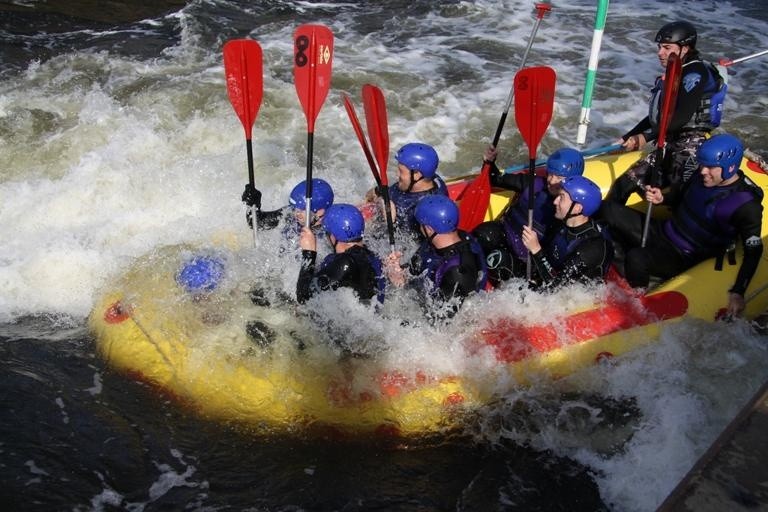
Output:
[654,20,698,49]
[394,142,439,179]
[320,203,365,242]
[696,133,744,180]
[559,175,603,218]
[413,193,459,233]
[289,178,334,211]
[545,148,586,177]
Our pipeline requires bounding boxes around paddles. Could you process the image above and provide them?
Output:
[224,39,263,248]
[342,95,381,187]
[293,24,334,228]
[442,144,625,183]
[458,4,552,232]
[642,52,683,247]
[514,65,556,281]
[362,84,394,253]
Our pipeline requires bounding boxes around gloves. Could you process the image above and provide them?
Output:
[241,184,262,209]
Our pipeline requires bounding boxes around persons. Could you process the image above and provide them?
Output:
[368,143,478,322]
[588,133,764,324]
[483,146,610,287]
[242,179,386,348]
[605,19,724,220]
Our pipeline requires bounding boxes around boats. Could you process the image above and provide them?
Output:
[84,131,768,440]
